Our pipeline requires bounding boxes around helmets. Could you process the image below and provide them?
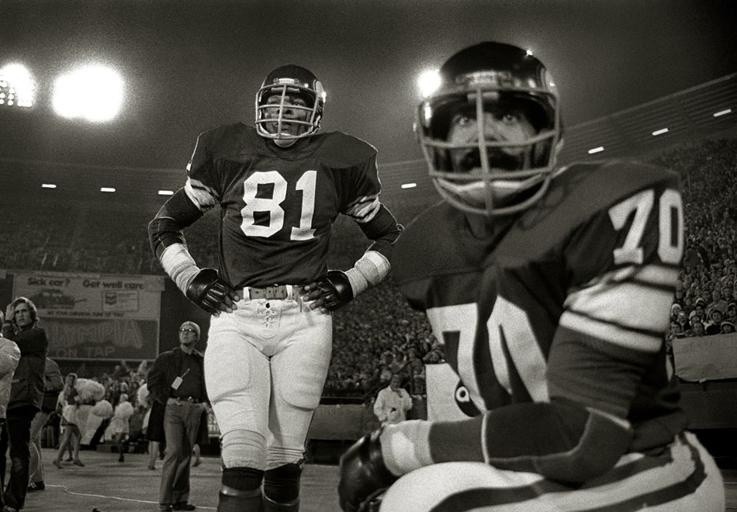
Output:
[256,64,326,147]
[413,42,566,216]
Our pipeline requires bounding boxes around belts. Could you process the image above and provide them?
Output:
[171,396,202,403]
[235,283,302,300]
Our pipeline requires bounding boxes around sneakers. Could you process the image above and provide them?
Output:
[193,460,202,467]
[26,481,44,491]
[53,457,84,469]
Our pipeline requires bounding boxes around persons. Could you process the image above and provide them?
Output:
[337,42,727,511]
[372,372,413,424]
[0,294,212,512]
[326,126,737,404]
[146,62,407,512]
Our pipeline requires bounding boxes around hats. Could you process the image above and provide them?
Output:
[178,321,201,344]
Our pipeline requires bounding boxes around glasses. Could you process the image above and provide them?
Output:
[179,327,196,335]
